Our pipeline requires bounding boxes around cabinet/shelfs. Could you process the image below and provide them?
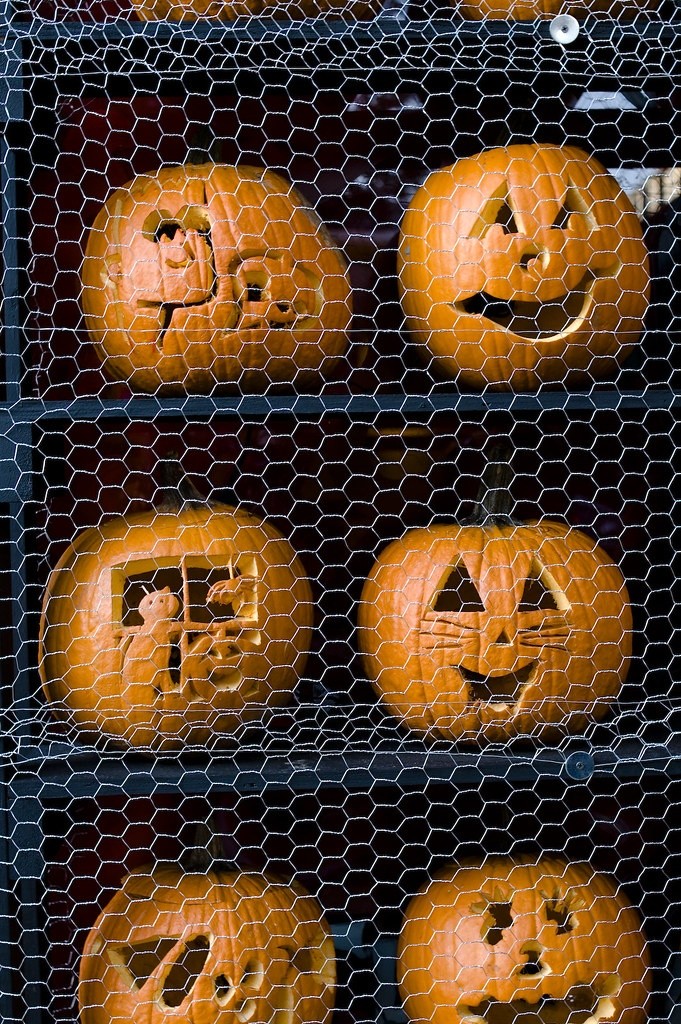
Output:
[0,1,681,1024]
